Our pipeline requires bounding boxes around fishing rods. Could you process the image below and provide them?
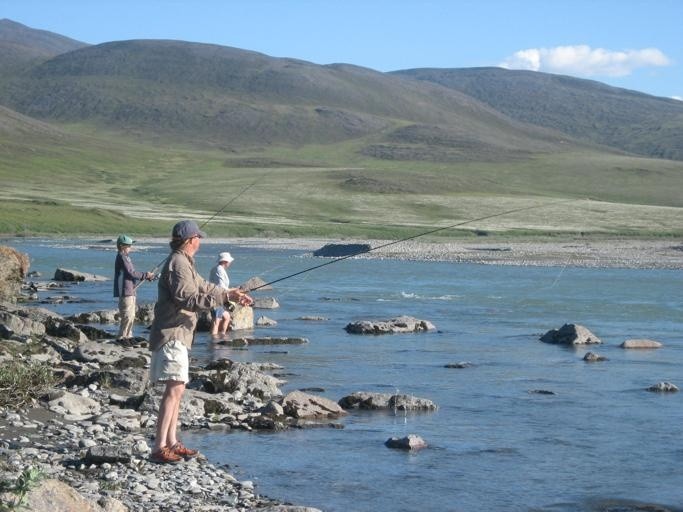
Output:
[136,167,276,291]
[245,198,584,296]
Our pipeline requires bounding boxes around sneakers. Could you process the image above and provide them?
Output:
[169,442,199,457]
[117,336,141,349]
[149,446,185,464]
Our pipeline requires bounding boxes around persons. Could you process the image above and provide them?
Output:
[208,251,235,335]
[149,219,253,464]
[113,236,153,342]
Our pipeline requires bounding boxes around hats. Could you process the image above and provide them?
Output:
[117,235,137,247]
[216,251,235,264]
[171,220,208,242]
